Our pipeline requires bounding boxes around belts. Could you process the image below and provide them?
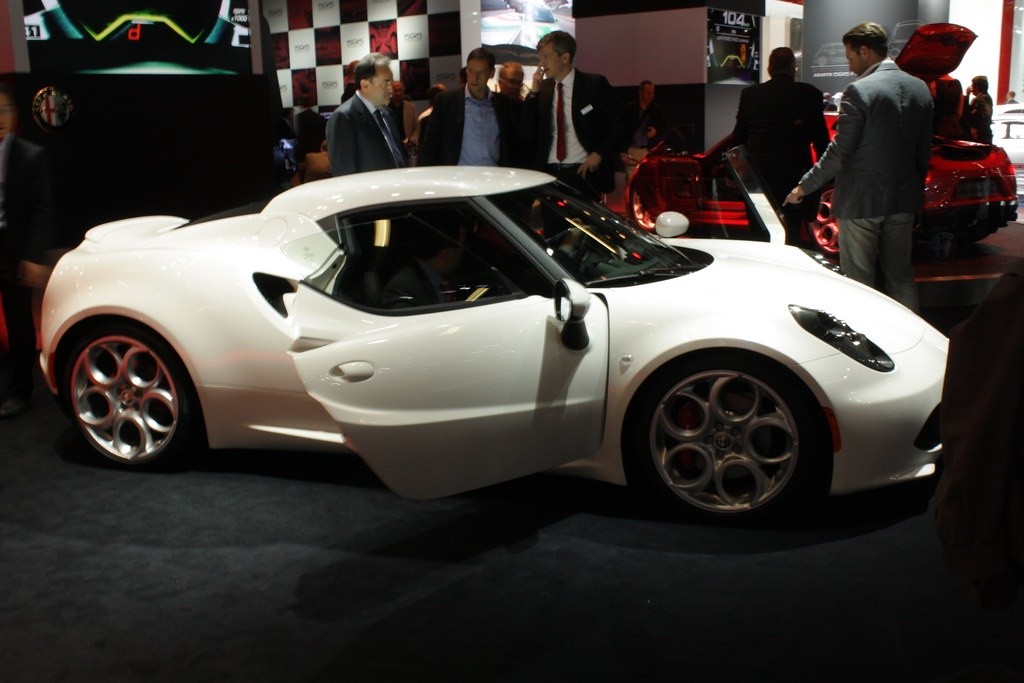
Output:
[548,164,579,171]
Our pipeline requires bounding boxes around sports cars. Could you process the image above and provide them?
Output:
[36,167,953,524]
[614,19,1020,269]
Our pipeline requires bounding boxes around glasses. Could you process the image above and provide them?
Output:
[367,80,392,86]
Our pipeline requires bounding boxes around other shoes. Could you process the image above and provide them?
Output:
[0,398,26,420]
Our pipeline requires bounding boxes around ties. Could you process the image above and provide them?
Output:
[556,82,567,163]
[374,109,407,168]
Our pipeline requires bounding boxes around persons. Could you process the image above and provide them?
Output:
[0,91,53,416]
[418,30,616,202]
[704,47,830,248]
[781,24,934,314]
[623,80,667,147]
[370,32,398,59]
[946,76,993,144]
[284,52,420,181]
[823,102,836,111]
[1006,91,1018,104]
[381,206,540,309]
